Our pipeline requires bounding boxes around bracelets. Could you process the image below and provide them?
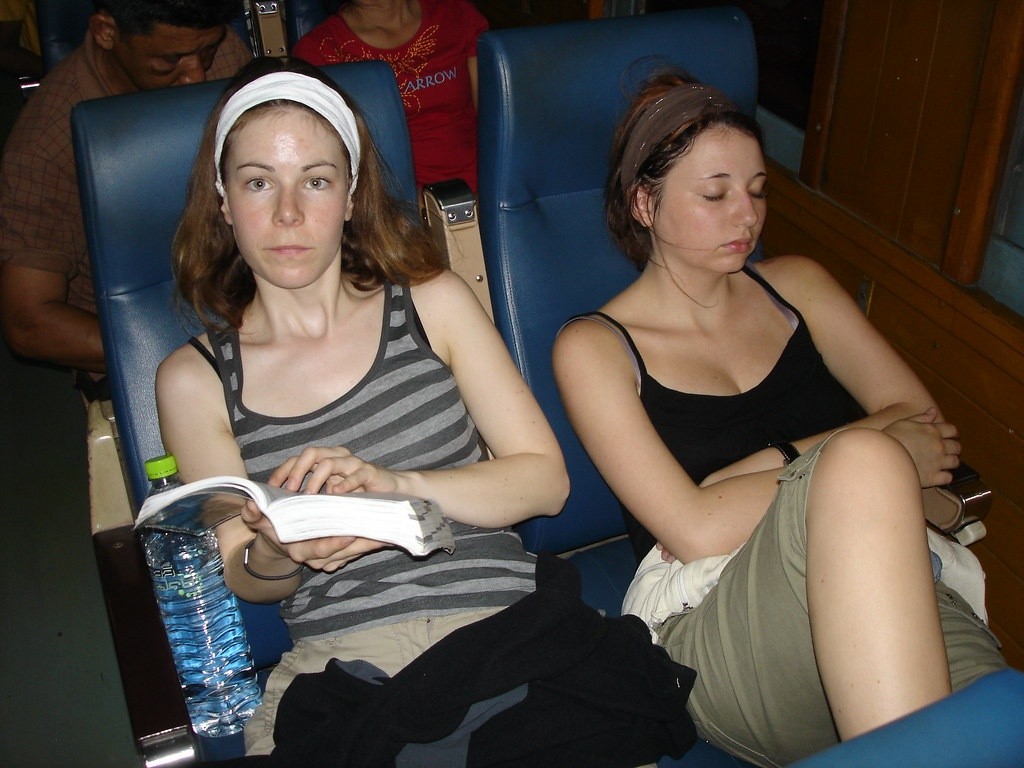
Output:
[244,538,304,580]
[768,441,799,466]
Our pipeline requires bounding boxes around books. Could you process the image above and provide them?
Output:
[131,475,456,558]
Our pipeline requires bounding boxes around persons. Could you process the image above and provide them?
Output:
[148,60,570,768]
[295,0,494,202]
[0,0,259,382]
[551,56,1011,768]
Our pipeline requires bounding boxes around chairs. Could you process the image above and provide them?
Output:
[283,0,345,57]
[475,7,772,768]
[35,0,291,79]
[69,62,551,768]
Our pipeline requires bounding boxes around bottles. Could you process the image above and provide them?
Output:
[139,456,263,737]
